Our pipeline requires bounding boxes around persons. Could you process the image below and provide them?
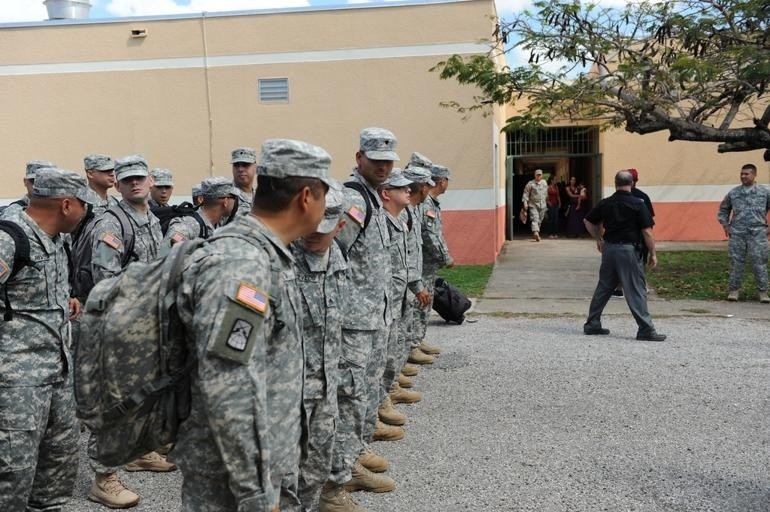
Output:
[716,164,770,303]
[215,145,257,228]
[547,175,561,239]
[148,167,174,211]
[318,419,396,512]
[521,169,548,241]
[396,166,436,388]
[86,155,175,508]
[190,184,204,208]
[575,183,587,210]
[176,138,341,512]
[409,165,454,365]
[164,175,242,244]
[0,167,96,512]
[565,176,587,238]
[64,152,120,242]
[583,169,667,341]
[0,161,59,214]
[319,126,405,472]
[378,167,423,425]
[282,174,350,511]
[599,168,656,299]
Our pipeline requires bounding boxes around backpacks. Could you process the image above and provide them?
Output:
[71,236,203,463]
[71,204,134,300]
[150,201,209,240]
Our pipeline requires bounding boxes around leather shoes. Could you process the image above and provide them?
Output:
[637,330,666,341]
[584,325,610,335]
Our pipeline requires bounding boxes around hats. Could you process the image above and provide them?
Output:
[114,155,149,181]
[431,165,454,180]
[406,167,436,187]
[192,184,202,197]
[150,168,174,186]
[84,153,114,170]
[256,139,343,191]
[628,169,638,177]
[32,168,100,204]
[535,169,542,175]
[360,128,399,161]
[315,180,345,233]
[26,161,54,178]
[201,177,241,196]
[230,148,255,164]
[380,169,414,186]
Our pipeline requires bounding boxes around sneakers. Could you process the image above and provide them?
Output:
[611,290,623,298]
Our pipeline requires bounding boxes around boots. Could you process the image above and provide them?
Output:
[319,483,372,512]
[378,397,406,425]
[388,382,421,403]
[358,446,387,472]
[407,348,433,363]
[126,452,178,473]
[728,289,740,301]
[401,367,419,376]
[395,373,412,388]
[87,471,138,507]
[343,461,395,493]
[420,341,440,354]
[759,290,770,303]
[373,413,404,440]
